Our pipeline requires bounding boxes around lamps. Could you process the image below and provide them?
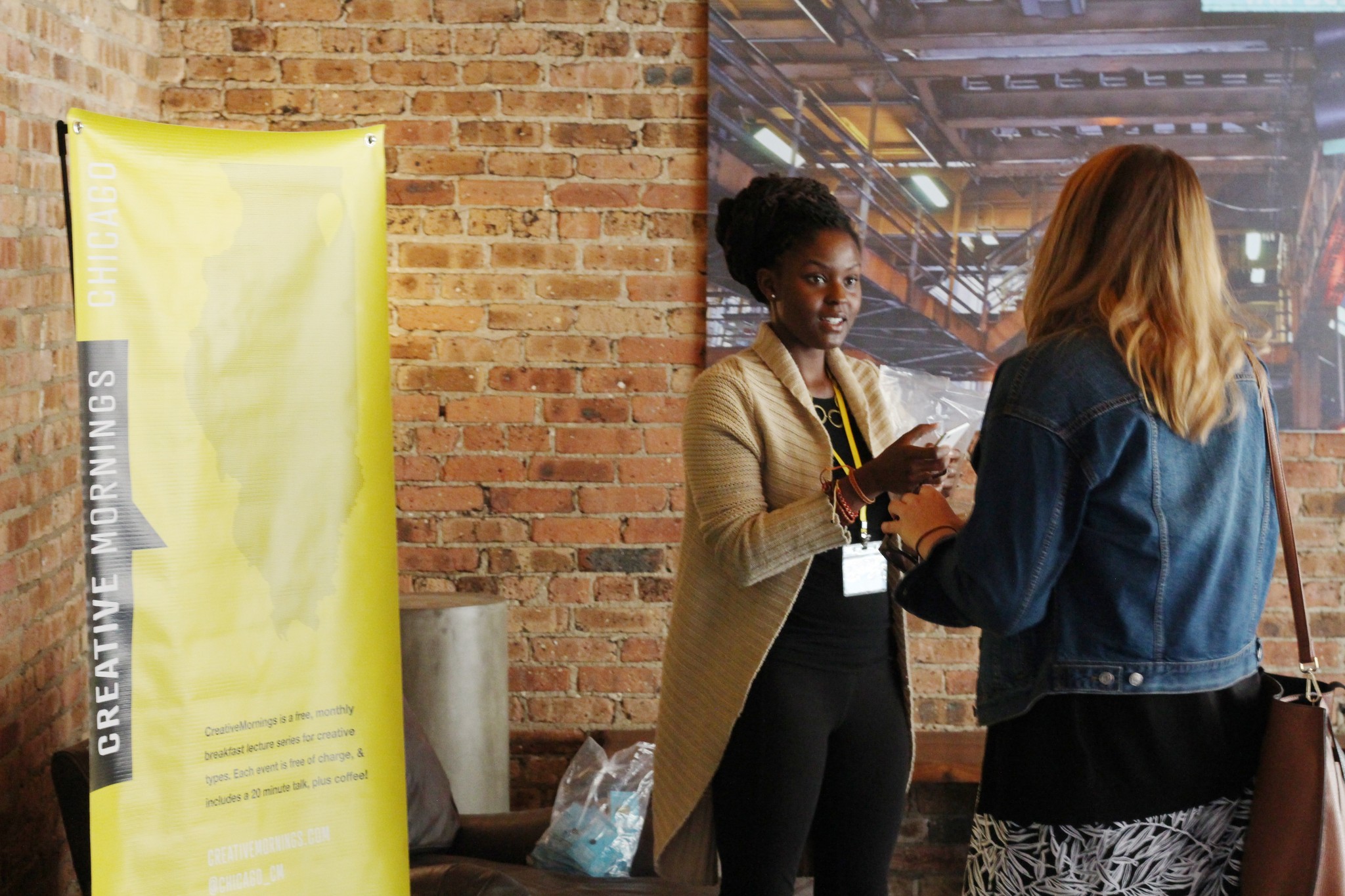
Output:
[752,123,806,167]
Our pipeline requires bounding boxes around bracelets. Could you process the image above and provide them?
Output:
[819,465,876,520]
[915,524,959,556]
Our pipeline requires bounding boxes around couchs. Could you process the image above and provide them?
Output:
[50,692,659,896]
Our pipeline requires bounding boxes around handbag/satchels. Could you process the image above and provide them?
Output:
[1237,673,1345,896]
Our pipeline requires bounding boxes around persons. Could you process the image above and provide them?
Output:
[652,172,977,895]
[880,146,1283,896]
